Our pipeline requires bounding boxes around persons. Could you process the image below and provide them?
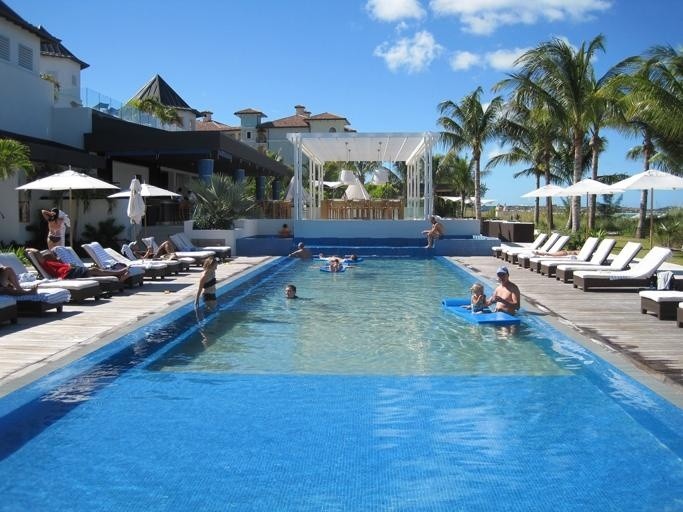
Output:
[279,223,291,238]
[318,254,357,273]
[501,325,518,337]
[531,248,577,256]
[0,266,38,296]
[285,284,298,299]
[28,248,130,281]
[195,255,218,311]
[462,266,520,315]
[174,188,195,220]
[288,242,310,258]
[196,310,219,349]
[471,284,486,315]
[129,241,177,260]
[40,208,64,251]
[421,217,445,248]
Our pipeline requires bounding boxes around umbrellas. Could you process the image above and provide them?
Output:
[521,168,683,249]
[106,179,182,238]
[127,174,146,239]
[15,164,121,247]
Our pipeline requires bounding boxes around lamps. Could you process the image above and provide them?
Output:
[340,139,389,187]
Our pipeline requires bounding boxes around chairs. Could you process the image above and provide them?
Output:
[258,197,407,221]
[0,233,232,330]
[492,232,683,329]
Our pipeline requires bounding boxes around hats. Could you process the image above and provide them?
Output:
[497,266,507,274]
